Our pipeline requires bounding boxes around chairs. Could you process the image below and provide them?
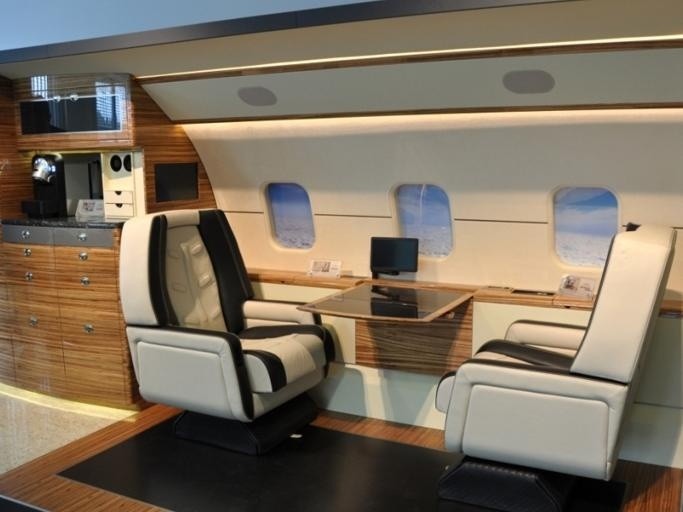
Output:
[117,207,334,457]
[432,223,676,512]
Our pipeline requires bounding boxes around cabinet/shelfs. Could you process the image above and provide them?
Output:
[0,226,157,421]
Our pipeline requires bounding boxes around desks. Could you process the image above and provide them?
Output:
[294,282,473,380]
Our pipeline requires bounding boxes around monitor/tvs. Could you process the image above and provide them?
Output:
[370,236,419,277]
[371,286,418,317]
